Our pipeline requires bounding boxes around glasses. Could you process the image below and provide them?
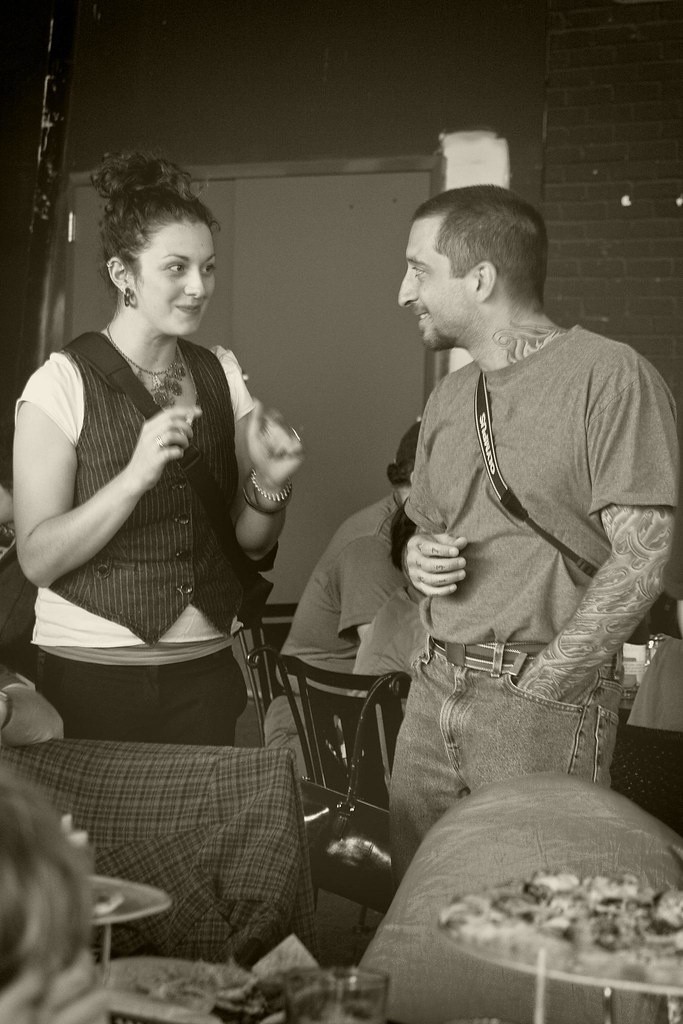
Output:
[0,523,17,548]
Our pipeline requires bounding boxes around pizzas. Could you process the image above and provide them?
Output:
[436,867,682,992]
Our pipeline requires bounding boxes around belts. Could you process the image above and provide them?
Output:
[431,636,618,677]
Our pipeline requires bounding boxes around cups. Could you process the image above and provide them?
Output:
[284,966,390,1024]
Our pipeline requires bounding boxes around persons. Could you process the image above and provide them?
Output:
[0,432,64,748]
[264,420,428,799]
[13,152,305,745]
[0,771,113,1024]
[388,185,678,898]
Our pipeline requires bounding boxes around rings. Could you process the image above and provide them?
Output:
[289,426,302,443]
[154,435,168,448]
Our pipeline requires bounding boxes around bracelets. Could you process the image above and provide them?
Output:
[240,468,293,515]
[0,690,13,730]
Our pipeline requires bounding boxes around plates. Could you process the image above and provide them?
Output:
[100,957,257,1017]
[439,880,682,997]
[89,872,171,925]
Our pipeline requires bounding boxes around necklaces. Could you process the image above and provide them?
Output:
[107,322,187,410]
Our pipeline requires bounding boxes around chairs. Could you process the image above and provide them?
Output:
[245,648,411,928]
[237,603,297,748]
